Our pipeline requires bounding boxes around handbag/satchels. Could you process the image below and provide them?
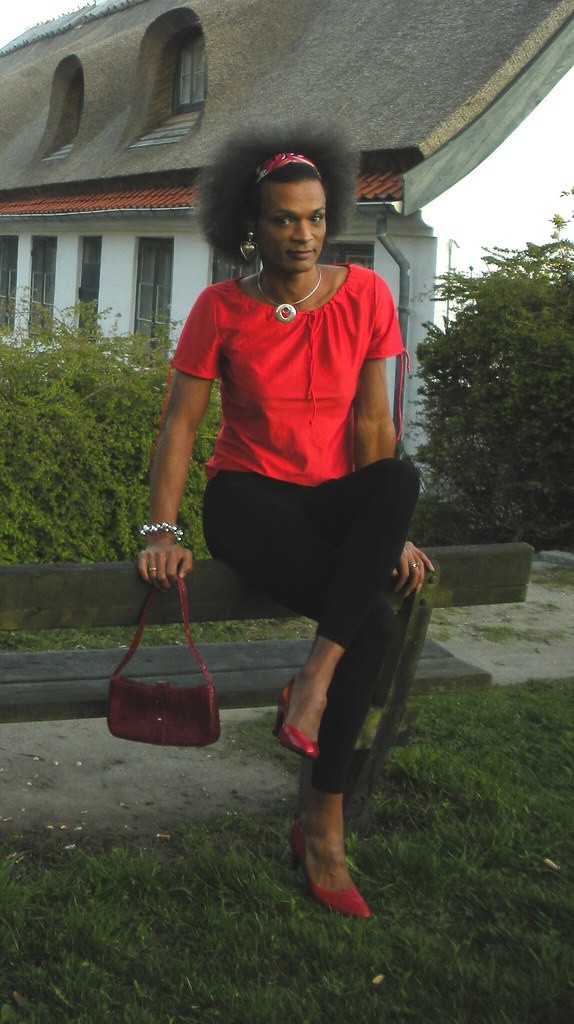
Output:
[107,576,221,746]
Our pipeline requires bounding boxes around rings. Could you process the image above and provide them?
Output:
[148,567,157,572]
[409,562,420,568]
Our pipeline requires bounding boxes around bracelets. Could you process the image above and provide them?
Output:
[140,522,184,544]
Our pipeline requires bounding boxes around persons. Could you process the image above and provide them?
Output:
[136,119,436,920]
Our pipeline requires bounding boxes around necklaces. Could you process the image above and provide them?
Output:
[257,263,322,323]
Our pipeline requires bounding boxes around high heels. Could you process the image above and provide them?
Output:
[288,818,372,918]
[273,677,320,762]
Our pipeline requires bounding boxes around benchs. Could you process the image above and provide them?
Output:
[1,541,535,820]
